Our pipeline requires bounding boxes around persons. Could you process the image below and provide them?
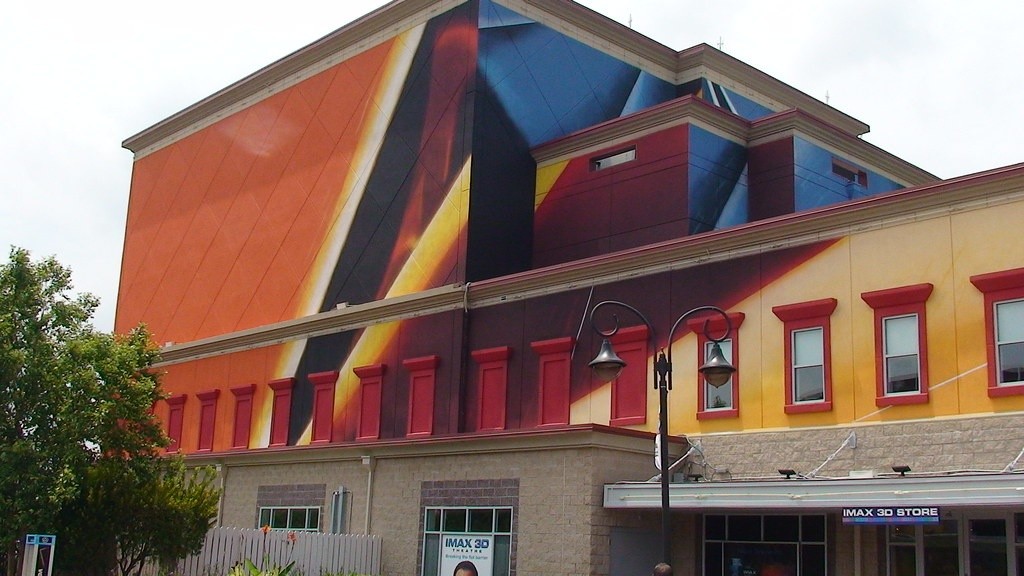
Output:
[652,563,671,576]
[453,560,478,576]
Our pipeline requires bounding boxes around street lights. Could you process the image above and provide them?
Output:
[585,300,737,575]
